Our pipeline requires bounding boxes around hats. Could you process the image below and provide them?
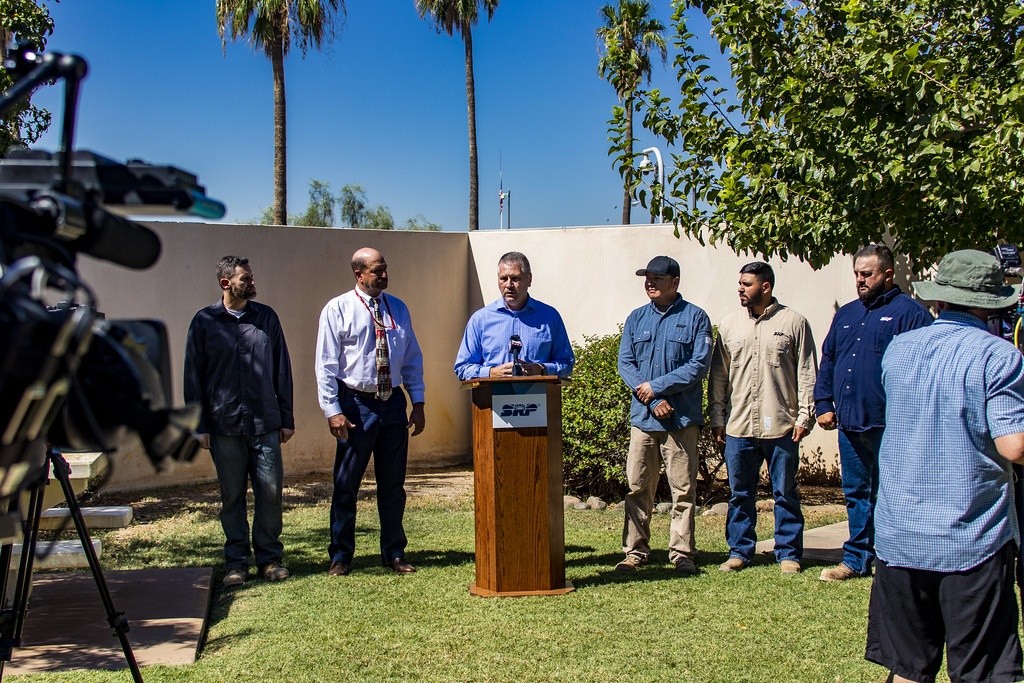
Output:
[911,249,1022,309]
[636,256,680,278]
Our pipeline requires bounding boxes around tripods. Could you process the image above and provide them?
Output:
[0,446,143,683]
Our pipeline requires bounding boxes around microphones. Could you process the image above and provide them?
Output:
[37,192,162,272]
[508,334,523,375]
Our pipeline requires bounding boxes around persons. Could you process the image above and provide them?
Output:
[707,261,818,573]
[315,246,425,576]
[864,247,1024,683]
[183,255,295,588]
[615,256,714,573]
[454,252,575,381]
[813,244,937,581]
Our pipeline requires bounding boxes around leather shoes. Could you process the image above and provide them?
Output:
[328,561,352,576]
[382,556,415,572]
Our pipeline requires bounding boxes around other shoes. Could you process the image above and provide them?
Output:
[718,558,745,572]
[674,557,698,576]
[615,556,642,572]
[819,562,855,581]
[257,559,289,580]
[223,568,246,587]
[780,560,800,574]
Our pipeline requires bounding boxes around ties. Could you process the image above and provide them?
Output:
[370,298,392,402]
